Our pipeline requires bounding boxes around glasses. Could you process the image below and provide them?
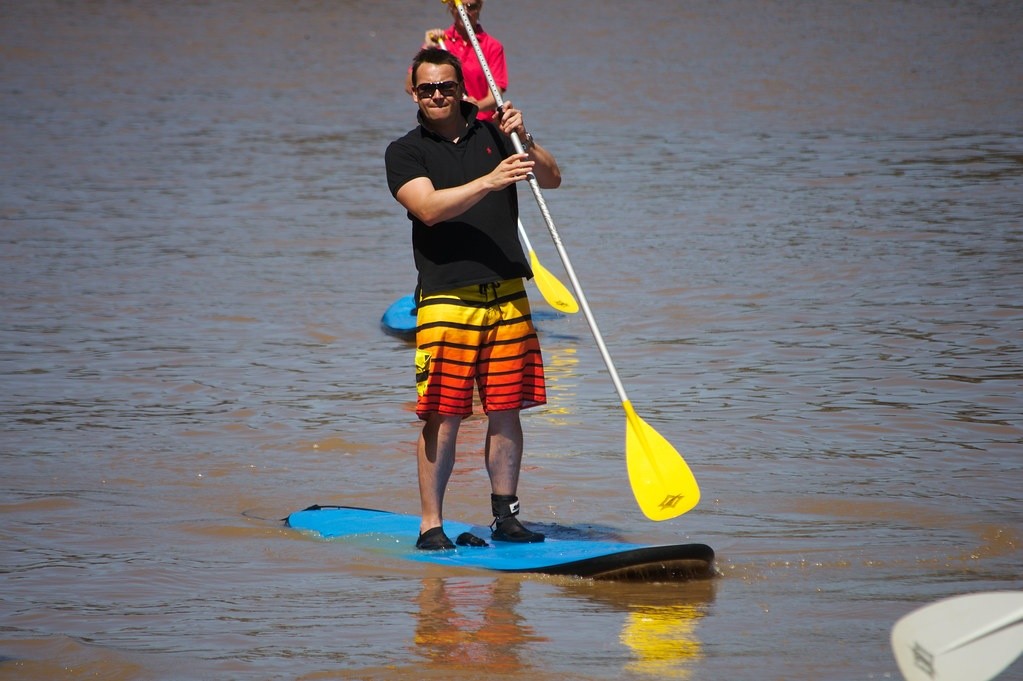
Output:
[453,3,478,11]
[415,80,460,98]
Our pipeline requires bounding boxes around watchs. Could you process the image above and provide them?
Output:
[521,132,533,149]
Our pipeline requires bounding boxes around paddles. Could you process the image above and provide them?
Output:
[441,1,703,523]
[429,32,581,315]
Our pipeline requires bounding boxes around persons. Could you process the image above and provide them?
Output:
[405,0,508,123]
[385,47,562,549]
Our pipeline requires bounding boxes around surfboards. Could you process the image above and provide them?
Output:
[886,586,1023,681]
[377,292,417,335]
[281,503,716,582]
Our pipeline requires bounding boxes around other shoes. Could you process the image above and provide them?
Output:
[414,527,457,549]
[490,517,545,543]
